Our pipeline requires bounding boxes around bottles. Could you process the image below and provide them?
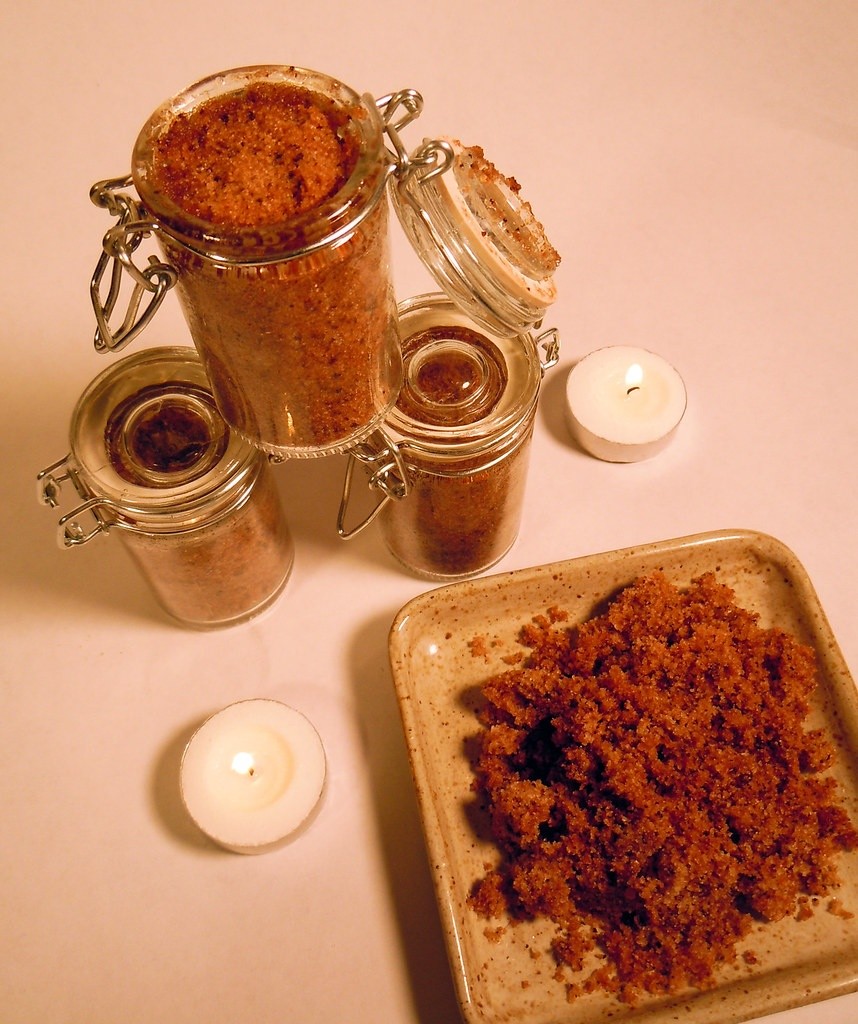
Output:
[337,291,560,583]
[35,345,294,630]
[89,62,561,459]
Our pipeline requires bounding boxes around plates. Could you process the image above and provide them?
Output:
[389,528,858,1024]
